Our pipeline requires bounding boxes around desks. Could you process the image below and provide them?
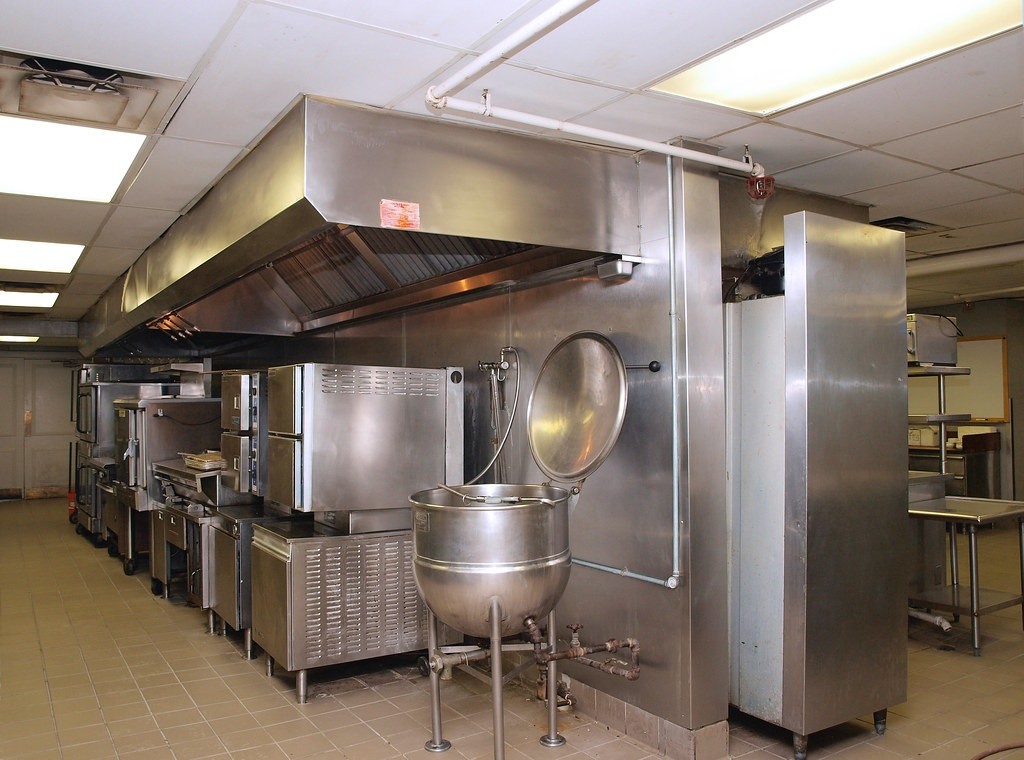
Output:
[909,496,1024,656]
